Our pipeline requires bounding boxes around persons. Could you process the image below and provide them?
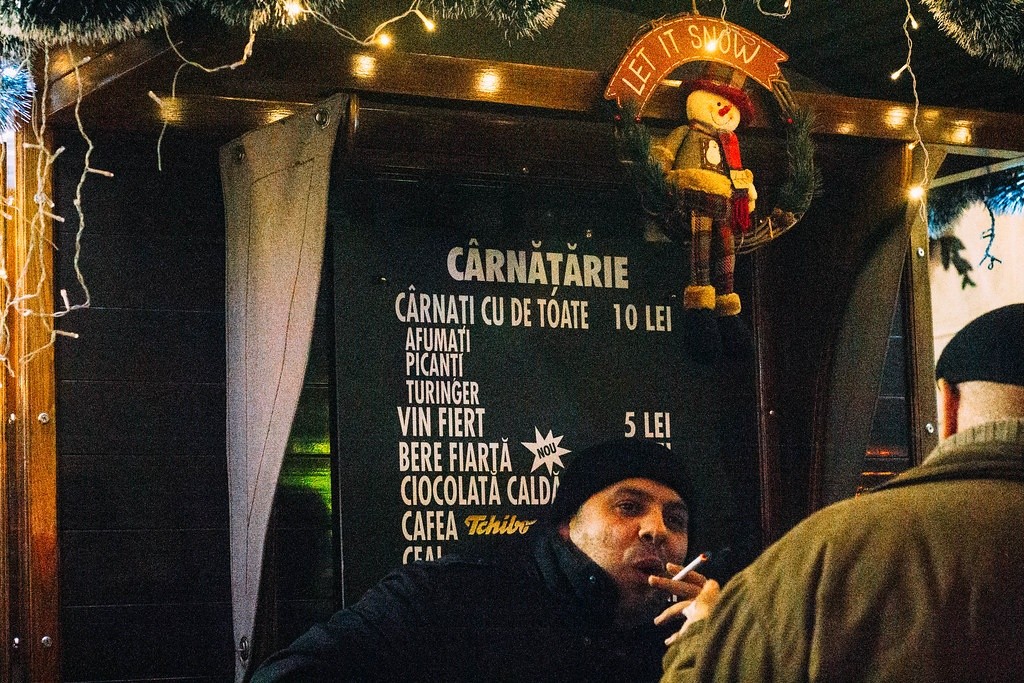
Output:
[247,438,719,683]
[659,302,1023,682]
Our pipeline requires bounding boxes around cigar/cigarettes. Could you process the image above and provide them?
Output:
[671,552,707,582]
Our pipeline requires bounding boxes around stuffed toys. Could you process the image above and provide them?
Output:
[647,76,762,360]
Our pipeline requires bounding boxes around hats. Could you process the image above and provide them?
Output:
[930,298,1024,390]
[546,438,698,530]
